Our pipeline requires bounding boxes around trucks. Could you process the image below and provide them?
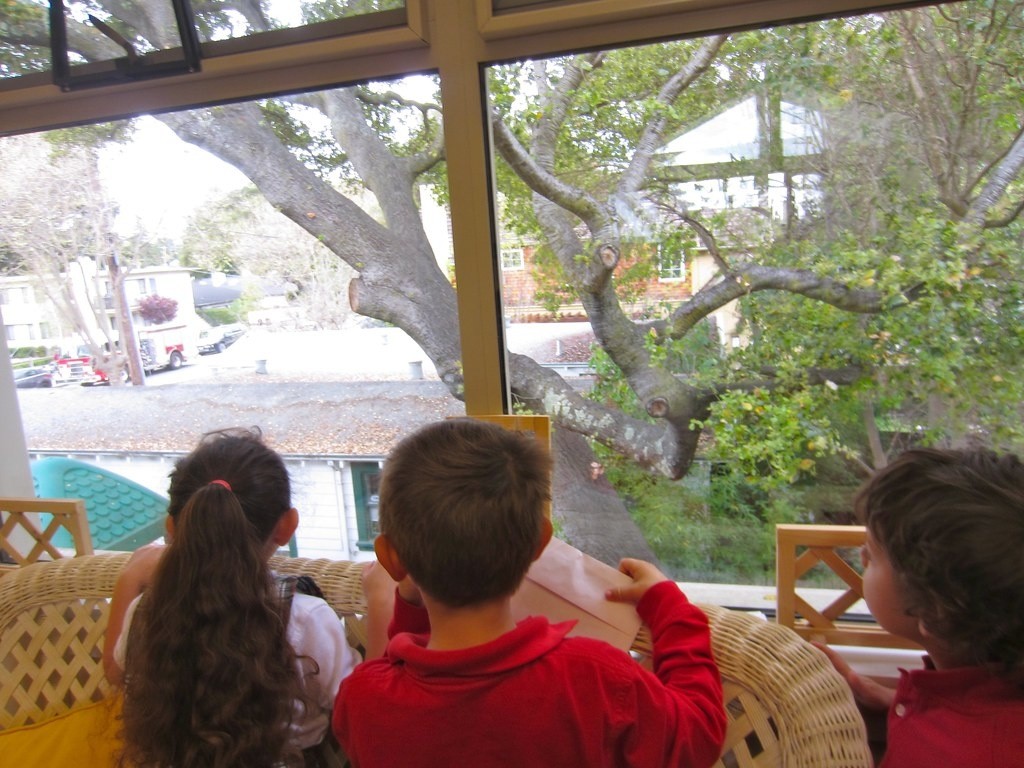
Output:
[55,331,187,388]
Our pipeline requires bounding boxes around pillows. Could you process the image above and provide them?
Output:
[0,690,159,768]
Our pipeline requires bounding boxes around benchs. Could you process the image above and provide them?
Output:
[0,553,877,768]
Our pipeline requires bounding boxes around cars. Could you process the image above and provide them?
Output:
[14,367,54,389]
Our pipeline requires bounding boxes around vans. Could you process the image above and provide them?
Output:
[197,323,245,357]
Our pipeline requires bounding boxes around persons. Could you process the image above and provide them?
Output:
[329,418,726,767]
[809,445,1023,766]
[101,425,399,768]
